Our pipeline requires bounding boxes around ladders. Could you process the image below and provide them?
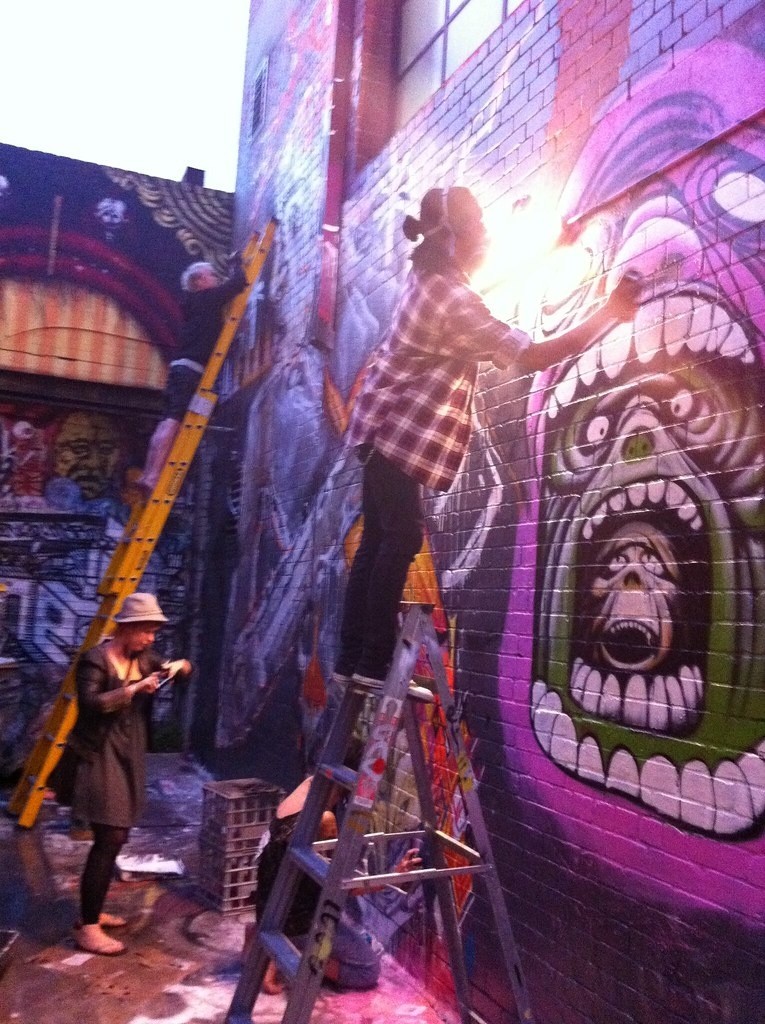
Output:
[9,217,277,827]
[227,603,533,1024]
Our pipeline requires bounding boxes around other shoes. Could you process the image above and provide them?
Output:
[240,921,260,958]
[264,961,283,994]
[353,661,433,700]
[332,661,349,684]
[73,922,130,956]
[100,915,131,926]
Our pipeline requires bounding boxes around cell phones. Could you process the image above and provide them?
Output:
[156,675,174,688]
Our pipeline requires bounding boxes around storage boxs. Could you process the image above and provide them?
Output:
[198,778,288,916]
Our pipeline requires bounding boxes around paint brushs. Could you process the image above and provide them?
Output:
[157,675,170,689]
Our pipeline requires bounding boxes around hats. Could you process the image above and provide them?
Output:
[113,592,170,622]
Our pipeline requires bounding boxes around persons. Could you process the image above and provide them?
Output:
[135,251,246,493]
[244,737,424,995]
[64,593,192,956]
[332,187,638,704]
